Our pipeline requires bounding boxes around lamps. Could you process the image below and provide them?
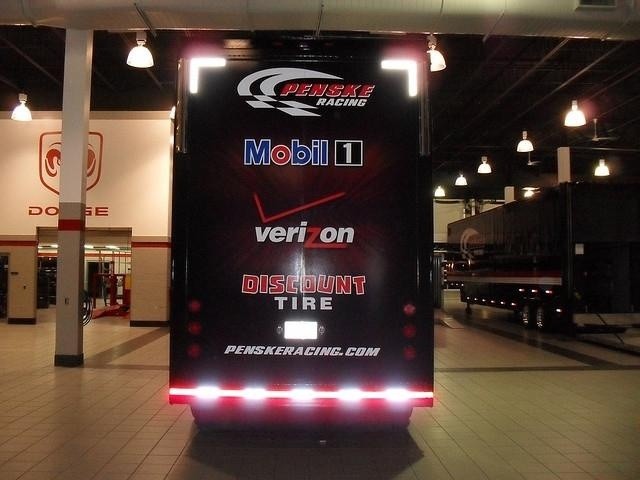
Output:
[434,173,467,198]
[477,157,492,174]
[594,160,609,176]
[564,101,586,127]
[517,131,534,152]
[11,94,32,121]
[427,35,446,72]
[126,32,154,68]
